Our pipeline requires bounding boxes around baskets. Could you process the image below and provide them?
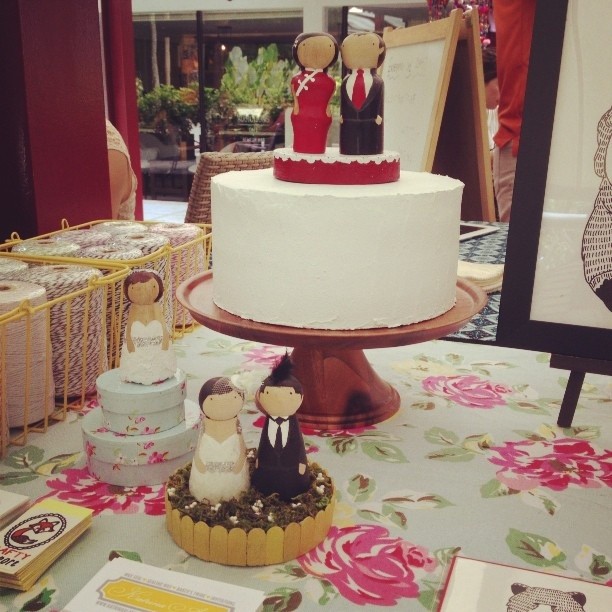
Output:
[1,217,213,344]
[1,233,129,461]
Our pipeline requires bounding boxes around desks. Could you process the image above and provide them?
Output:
[1,325,612,612]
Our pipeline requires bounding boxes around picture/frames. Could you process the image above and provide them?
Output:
[490,0,612,378]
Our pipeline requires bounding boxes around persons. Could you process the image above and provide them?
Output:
[492,1,537,223]
[482,48,501,168]
[106,117,138,221]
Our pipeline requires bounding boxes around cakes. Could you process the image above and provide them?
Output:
[208,30,465,329]
[80,271,203,487]
[166,355,335,566]
